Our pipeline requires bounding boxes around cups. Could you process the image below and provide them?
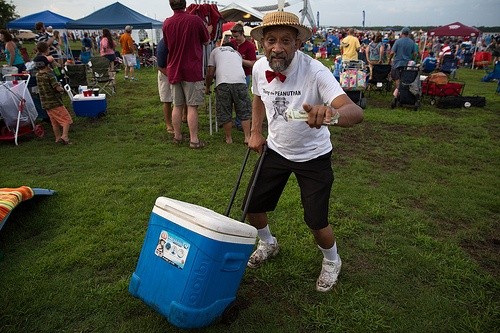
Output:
[83,88,99,97]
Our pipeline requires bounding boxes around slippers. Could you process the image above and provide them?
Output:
[225,137,233,143]
[167,128,175,134]
[60,137,72,145]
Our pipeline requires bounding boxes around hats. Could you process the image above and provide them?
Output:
[33,56,49,68]
[250,12,313,43]
[124,26,133,32]
[402,27,409,34]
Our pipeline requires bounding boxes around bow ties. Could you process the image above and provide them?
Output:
[265,70,286,83]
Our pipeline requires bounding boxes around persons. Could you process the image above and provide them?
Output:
[66,29,158,67]
[214,26,500,86]
[0,27,27,84]
[32,22,73,145]
[242,11,365,292]
[99,28,117,73]
[230,23,257,131]
[162,0,211,148]
[119,25,139,81]
[205,42,251,144]
[156,39,187,134]
[78,47,91,65]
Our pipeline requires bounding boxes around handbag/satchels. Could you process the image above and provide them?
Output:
[20,48,30,62]
[340,69,366,90]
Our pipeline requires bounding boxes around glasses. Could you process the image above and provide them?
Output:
[230,29,242,33]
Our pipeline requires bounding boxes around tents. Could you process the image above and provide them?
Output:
[66,2,163,71]
[218,1,265,50]
[420,22,479,69]
[6,10,75,61]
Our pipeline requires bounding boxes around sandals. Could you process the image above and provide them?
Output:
[172,137,185,144]
[190,140,205,149]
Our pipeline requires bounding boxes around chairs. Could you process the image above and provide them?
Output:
[441,58,458,81]
[65,65,95,93]
[421,59,437,74]
[70,50,81,64]
[91,57,117,98]
[366,64,392,98]
[139,49,154,68]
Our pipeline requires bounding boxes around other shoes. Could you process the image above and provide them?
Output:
[130,78,137,81]
[125,76,128,79]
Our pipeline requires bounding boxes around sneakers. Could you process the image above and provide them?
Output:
[317,255,341,291]
[248,237,278,268]
